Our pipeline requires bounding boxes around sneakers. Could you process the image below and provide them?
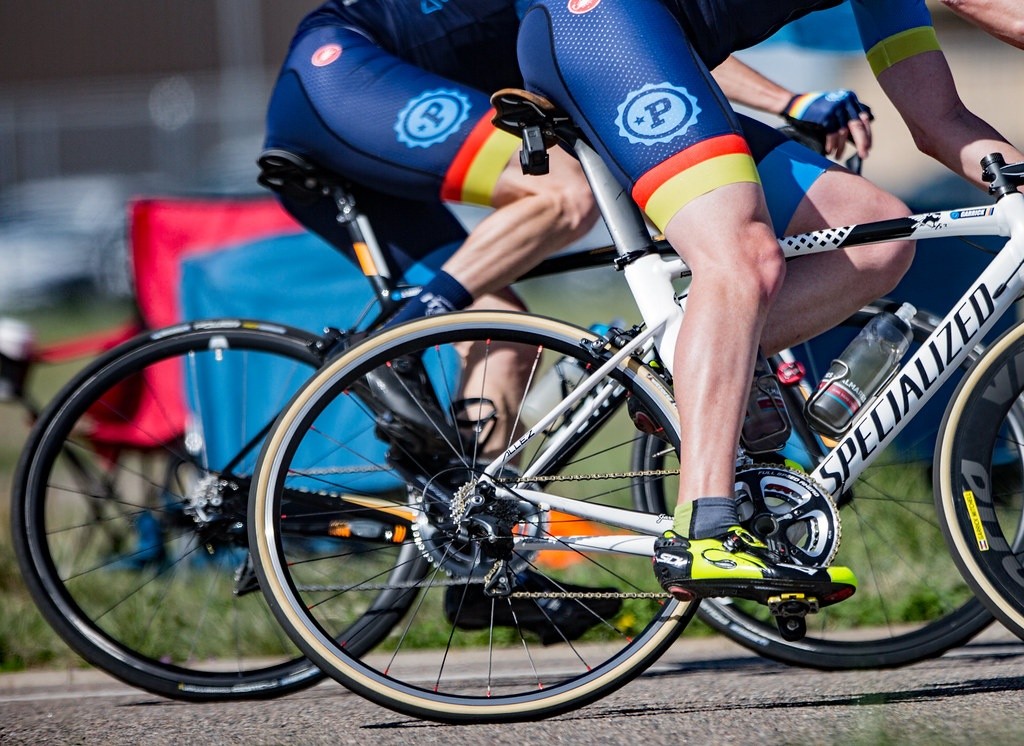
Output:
[651,525,856,608]
[443,567,623,640]
[626,360,804,469]
[325,333,452,439]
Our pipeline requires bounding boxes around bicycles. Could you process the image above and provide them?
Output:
[8,88,1024,725]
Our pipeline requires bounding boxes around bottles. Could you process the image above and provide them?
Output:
[803,301,918,438]
[519,319,627,433]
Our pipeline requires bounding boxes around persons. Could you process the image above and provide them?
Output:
[261,0,1024,629]
